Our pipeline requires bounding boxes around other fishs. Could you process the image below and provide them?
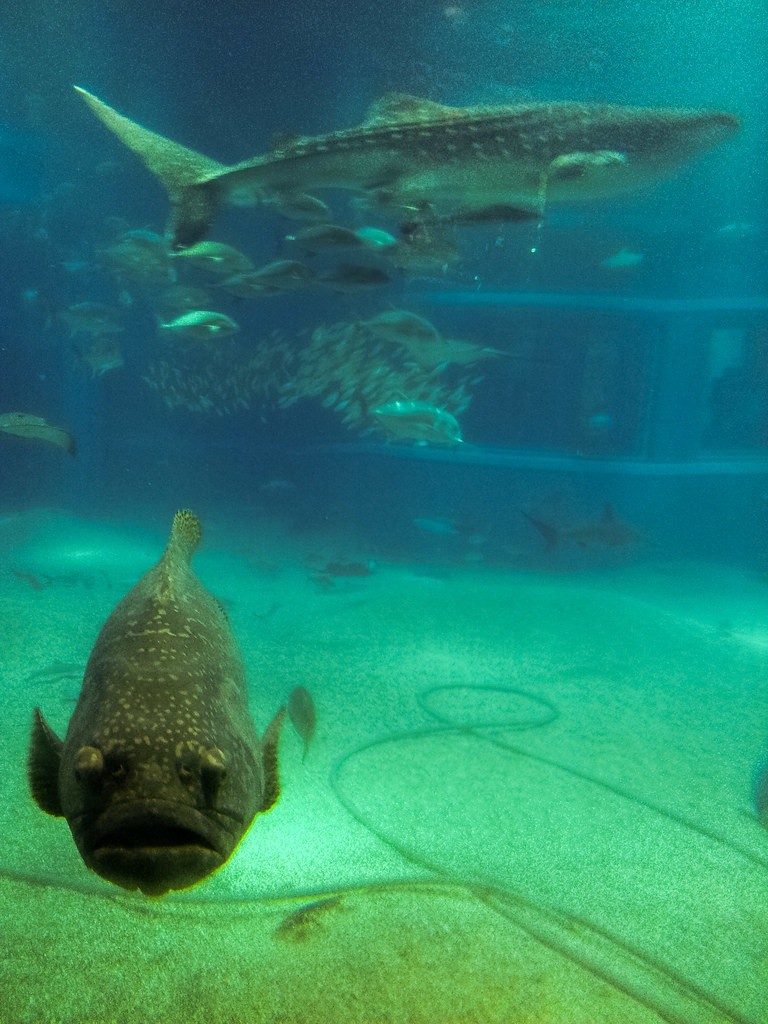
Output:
[24,505,285,899]
[0,215,746,453]
[518,502,667,570]
